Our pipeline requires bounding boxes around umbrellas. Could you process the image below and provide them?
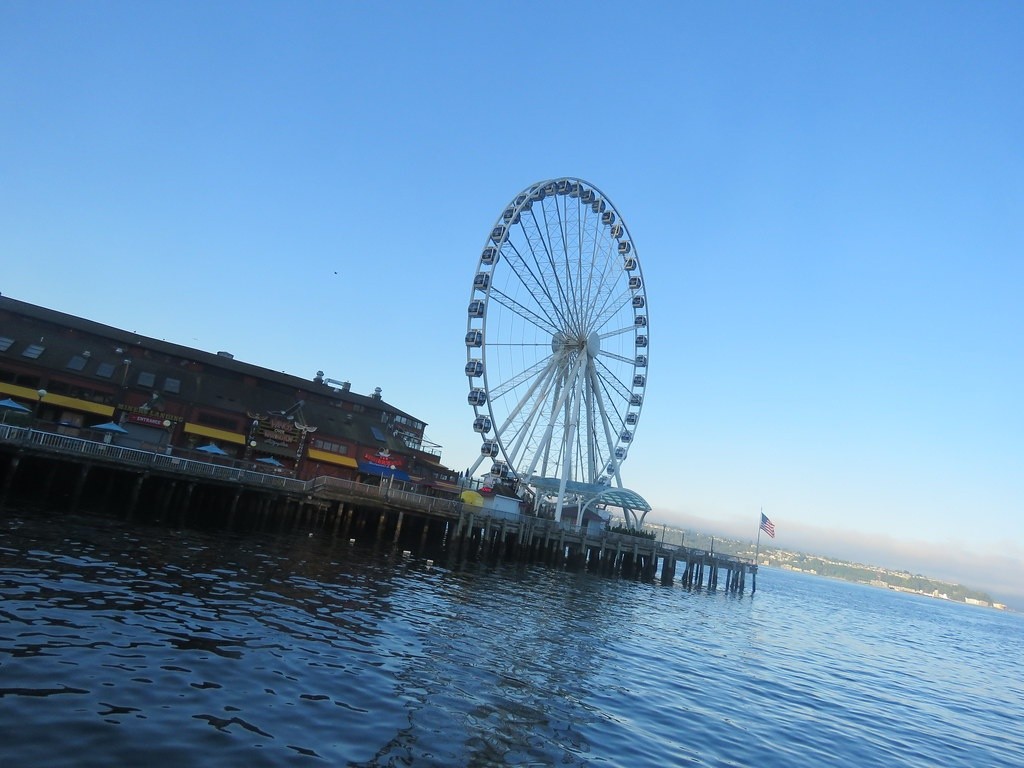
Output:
[256,456,284,467]
[0,398,32,424]
[56,419,81,433]
[90,421,128,444]
[196,444,229,463]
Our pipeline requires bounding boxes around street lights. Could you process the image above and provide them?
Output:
[156,420,170,451]
[661,524,666,548]
[245,441,257,470]
[29,389,47,430]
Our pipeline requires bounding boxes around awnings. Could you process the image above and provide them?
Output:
[0,382,116,417]
[306,448,358,469]
[184,422,245,444]
[358,460,409,480]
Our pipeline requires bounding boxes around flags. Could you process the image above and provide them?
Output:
[760,513,775,538]
